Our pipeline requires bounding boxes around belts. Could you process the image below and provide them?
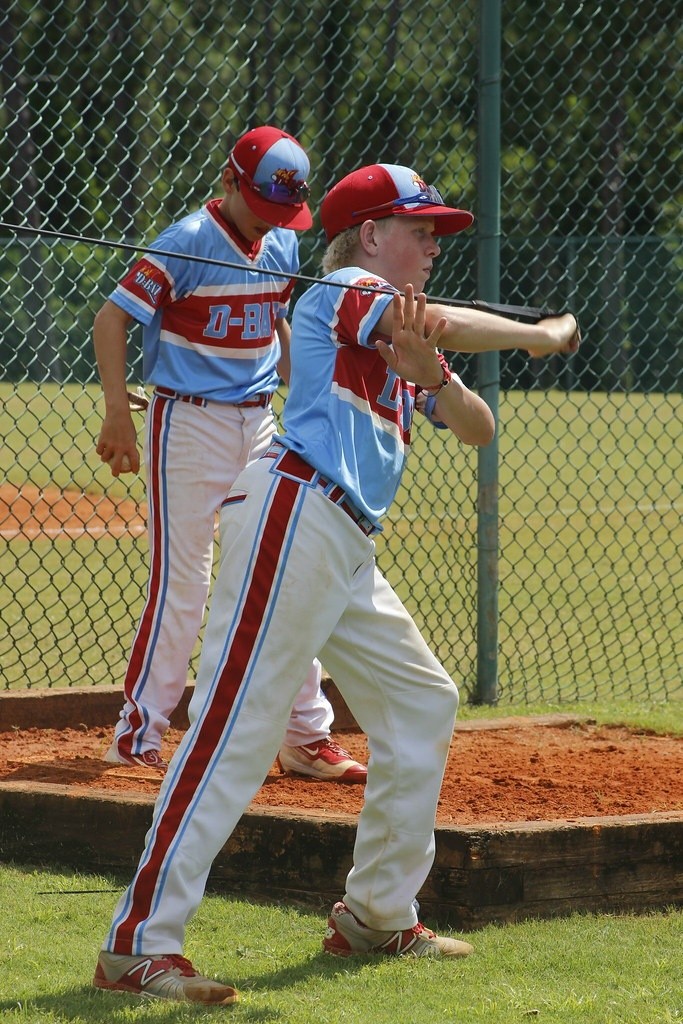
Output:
[259,442,380,538]
[154,385,276,408]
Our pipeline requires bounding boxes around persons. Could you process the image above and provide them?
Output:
[93,164,580,1005]
[92,122,368,784]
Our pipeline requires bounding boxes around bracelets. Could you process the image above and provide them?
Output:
[418,365,451,400]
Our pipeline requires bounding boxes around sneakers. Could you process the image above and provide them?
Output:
[322,902,474,963]
[105,744,169,769]
[277,737,367,782]
[93,950,240,1005]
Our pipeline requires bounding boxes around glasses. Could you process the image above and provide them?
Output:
[256,179,311,204]
[353,185,445,217]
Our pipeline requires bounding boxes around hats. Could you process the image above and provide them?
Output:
[321,164,474,243]
[228,126,313,230]
